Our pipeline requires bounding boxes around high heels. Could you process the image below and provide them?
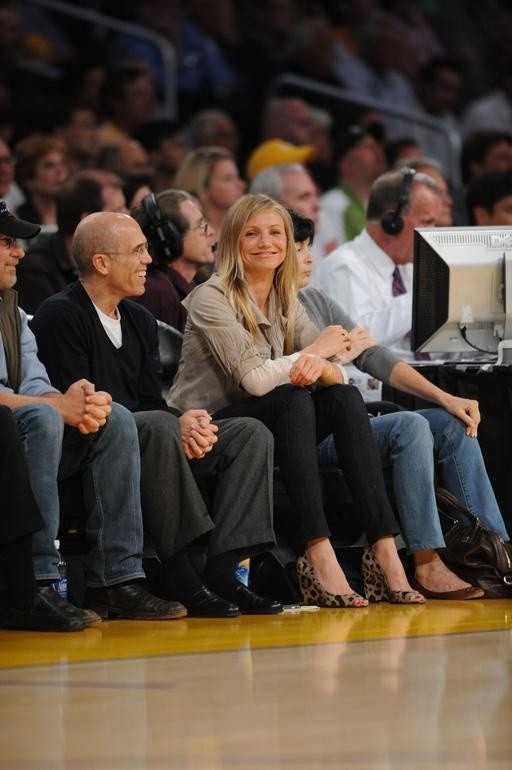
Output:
[361,546,423,604]
[295,546,369,607]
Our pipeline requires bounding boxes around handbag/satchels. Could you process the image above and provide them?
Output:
[434,486,512,599]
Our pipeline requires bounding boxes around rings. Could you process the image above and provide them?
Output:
[341,332,350,340]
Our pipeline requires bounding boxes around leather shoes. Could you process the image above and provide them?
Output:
[0,577,102,632]
[159,581,284,618]
[84,582,189,619]
[411,576,484,599]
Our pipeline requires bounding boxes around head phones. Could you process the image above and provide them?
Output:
[143,191,183,263]
[381,166,416,236]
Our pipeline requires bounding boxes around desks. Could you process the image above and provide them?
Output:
[381,360,512,573]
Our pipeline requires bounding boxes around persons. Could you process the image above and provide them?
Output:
[0,206,188,629]
[278,209,512,601]
[1,1,512,397]
[25,209,287,619]
[155,192,429,610]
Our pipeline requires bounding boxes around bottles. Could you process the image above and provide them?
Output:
[52,540,67,601]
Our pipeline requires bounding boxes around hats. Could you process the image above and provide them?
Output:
[1,201,41,239]
[244,138,316,181]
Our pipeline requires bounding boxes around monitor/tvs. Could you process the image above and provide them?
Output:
[410,225,512,353]
